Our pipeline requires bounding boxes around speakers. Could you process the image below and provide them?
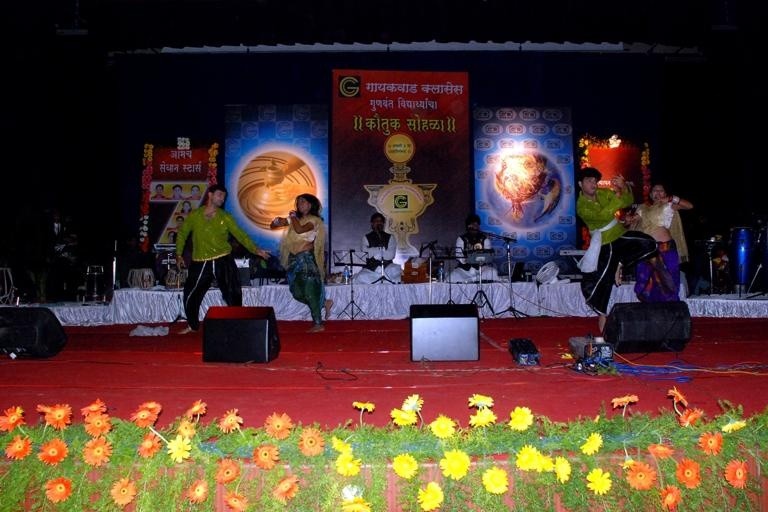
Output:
[600,299,693,352]
[409,303,480,361]
[0,306,68,359]
[203,306,280,363]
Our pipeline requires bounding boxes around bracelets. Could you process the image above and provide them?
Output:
[273,217,280,226]
[288,210,298,218]
[176,256,181,258]
[670,194,681,205]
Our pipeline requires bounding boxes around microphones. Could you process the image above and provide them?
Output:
[418,245,424,258]
[466,225,478,233]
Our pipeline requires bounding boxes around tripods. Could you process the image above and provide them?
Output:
[337,249,365,322]
[370,234,397,284]
[164,269,188,327]
[495,248,530,320]
[465,262,495,318]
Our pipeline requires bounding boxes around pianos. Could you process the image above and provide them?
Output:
[154,244,177,251]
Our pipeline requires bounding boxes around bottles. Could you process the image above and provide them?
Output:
[438,262,444,283]
[342,264,350,285]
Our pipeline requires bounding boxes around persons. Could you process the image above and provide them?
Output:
[575,166,659,333]
[151,185,202,245]
[624,184,695,302]
[270,193,334,334]
[353,212,403,285]
[117,235,148,288]
[445,214,498,283]
[175,185,272,334]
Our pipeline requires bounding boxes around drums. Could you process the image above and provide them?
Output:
[128,269,156,289]
[696,240,726,258]
[165,268,185,289]
[83,265,106,300]
[466,249,494,264]
[730,226,752,285]
